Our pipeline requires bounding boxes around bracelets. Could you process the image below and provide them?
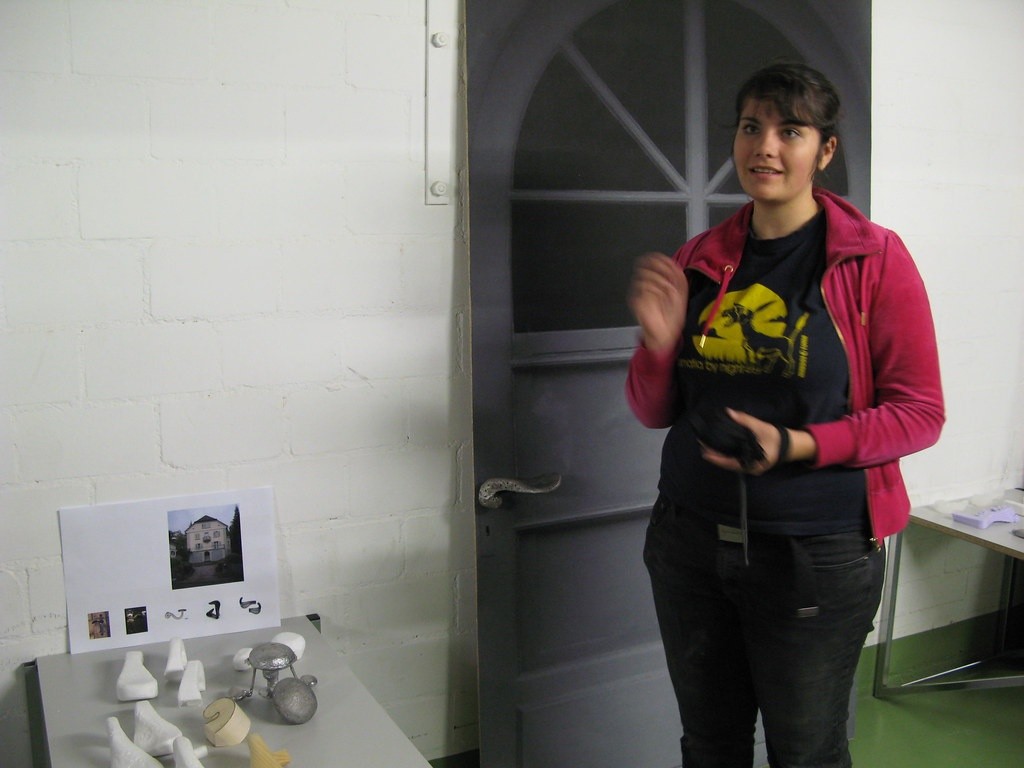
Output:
[775,424,789,461]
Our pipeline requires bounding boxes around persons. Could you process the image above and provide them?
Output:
[625,63,945,768]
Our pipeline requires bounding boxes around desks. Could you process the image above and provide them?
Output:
[23,613,433,768]
[873,486,1024,699]
